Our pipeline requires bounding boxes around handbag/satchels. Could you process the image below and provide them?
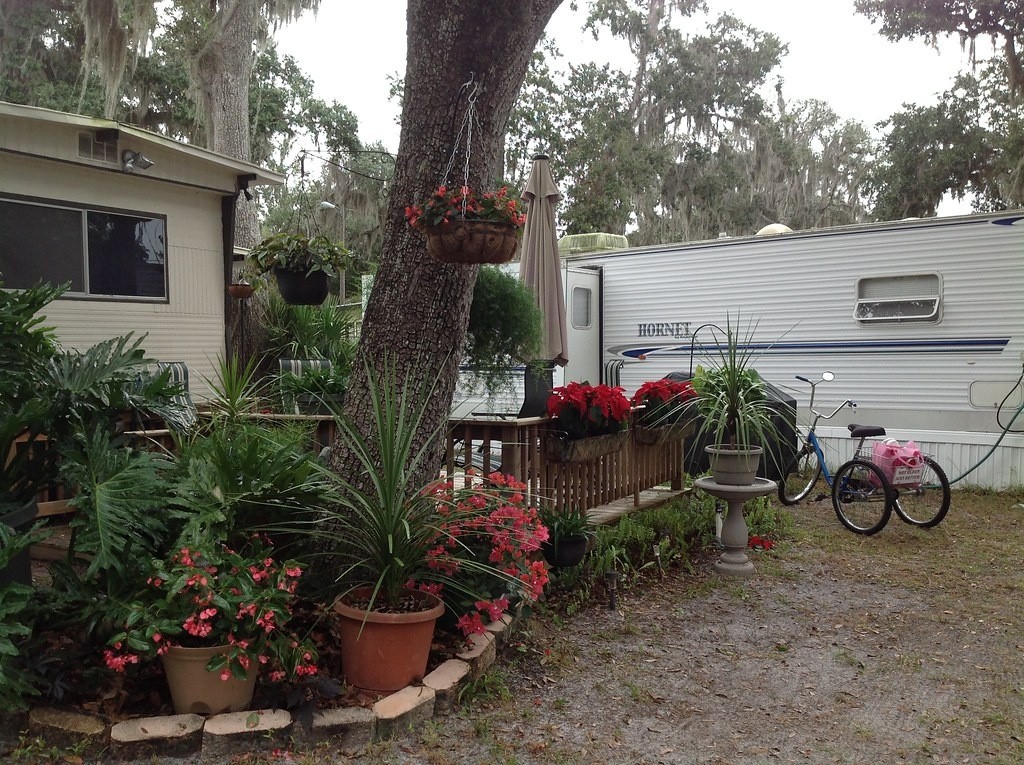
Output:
[868,440,923,486]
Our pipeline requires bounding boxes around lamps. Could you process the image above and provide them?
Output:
[243,189,253,202]
[122,148,155,174]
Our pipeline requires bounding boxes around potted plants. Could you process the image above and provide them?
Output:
[0,233,607,717]
[741,496,795,552]
[628,315,812,485]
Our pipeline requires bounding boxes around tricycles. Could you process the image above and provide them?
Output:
[778,371,951,535]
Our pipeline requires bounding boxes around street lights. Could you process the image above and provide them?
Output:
[320,201,346,305]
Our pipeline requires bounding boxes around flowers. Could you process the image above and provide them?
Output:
[406,468,550,644]
[104,545,319,685]
[630,378,698,420]
[545,383,631,430]
[403,181,528,242]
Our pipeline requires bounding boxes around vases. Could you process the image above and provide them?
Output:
[162,638,260,719]
[660,418,668,428]
[670,414,682,424]
[587,420,608,438]
[417,222,518,264]
[568,422,584,439]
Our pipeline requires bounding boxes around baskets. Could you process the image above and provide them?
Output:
[854,443,935,492]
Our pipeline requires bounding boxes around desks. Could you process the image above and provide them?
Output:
[694,477,777,577]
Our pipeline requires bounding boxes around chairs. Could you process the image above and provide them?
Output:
[472,359,557,453]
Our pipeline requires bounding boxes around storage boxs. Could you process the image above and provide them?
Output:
[869,441,924,488]
